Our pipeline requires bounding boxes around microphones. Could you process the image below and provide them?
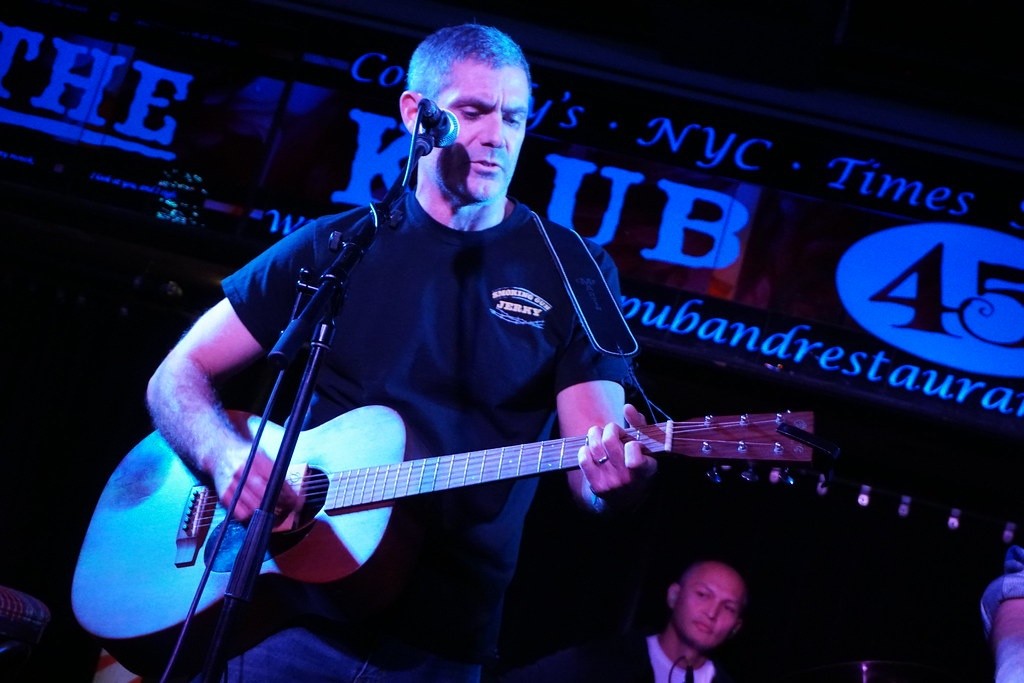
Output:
[420,98,460,148]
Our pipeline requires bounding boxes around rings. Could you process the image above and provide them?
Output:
[598,456,608,464]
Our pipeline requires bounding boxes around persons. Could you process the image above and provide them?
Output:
[500,560,749,683]
[980,545,1024,683]
[145,23,657,683]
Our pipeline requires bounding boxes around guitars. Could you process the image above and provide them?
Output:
[71,399,819,681]
